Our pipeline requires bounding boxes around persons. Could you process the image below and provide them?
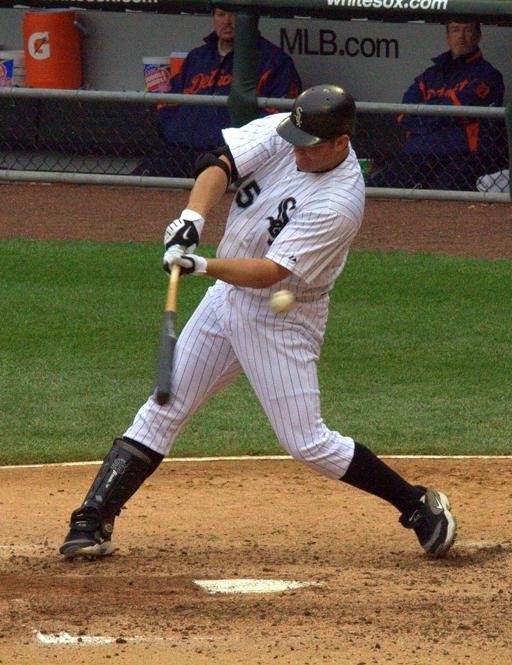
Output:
[130,4,302,177]
[58,84,457,559]
[365,11,505,190]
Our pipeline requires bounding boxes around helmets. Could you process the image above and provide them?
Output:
[276,82,359,149]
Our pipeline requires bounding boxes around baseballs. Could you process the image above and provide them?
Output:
[271,287,294,313]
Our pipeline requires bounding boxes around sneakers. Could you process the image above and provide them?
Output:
[397,485,459,560]
[59,506,118,561]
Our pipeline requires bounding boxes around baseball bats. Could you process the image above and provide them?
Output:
[155,262,181,405]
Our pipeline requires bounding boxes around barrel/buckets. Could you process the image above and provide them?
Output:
[357,159,377,183]
[140,56,172,93]
[475,172,510,194]
[168,51,191,79]
[1,59,14,86]
[22,10,79,88]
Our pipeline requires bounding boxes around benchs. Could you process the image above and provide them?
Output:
[0,85,506,188]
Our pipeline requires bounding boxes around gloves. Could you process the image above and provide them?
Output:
[161,243,209,277]
[163,209,205,252]
